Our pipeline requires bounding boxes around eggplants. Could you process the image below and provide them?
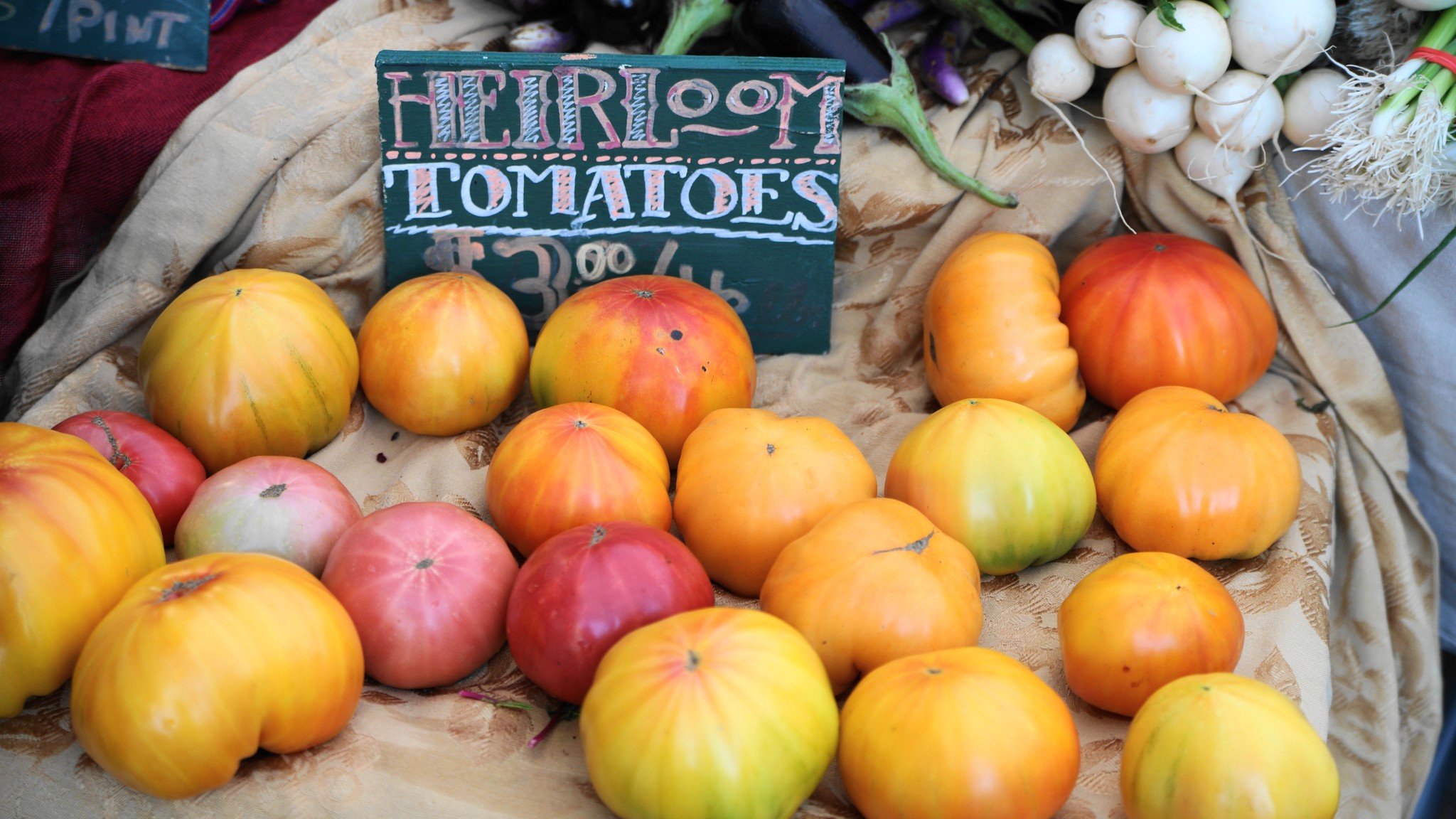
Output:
[480,0,999,230]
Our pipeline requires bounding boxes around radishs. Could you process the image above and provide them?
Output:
[1001,0,1371,244]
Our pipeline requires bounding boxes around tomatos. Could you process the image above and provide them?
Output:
[924,232,1085,438]
[485,399,676,560]
[574,592,843,819]
[1054,550,1245,715]
[138,270,356,476]
[1108,674,1335,817]
[522,277,758,463]
[881,393,1102,575]
[329,498,517,691]
[676,406,880,595]
[754,494,984,699]
[63,550,361,793]
[344,257,530,441]
[835,640,1083,819]
[1,418,172,716]
[500,508,718,711]
[1092,380,1302,567]
[1056,225,1278,401]
[175,448,356,567]
[46,404,195,533]
